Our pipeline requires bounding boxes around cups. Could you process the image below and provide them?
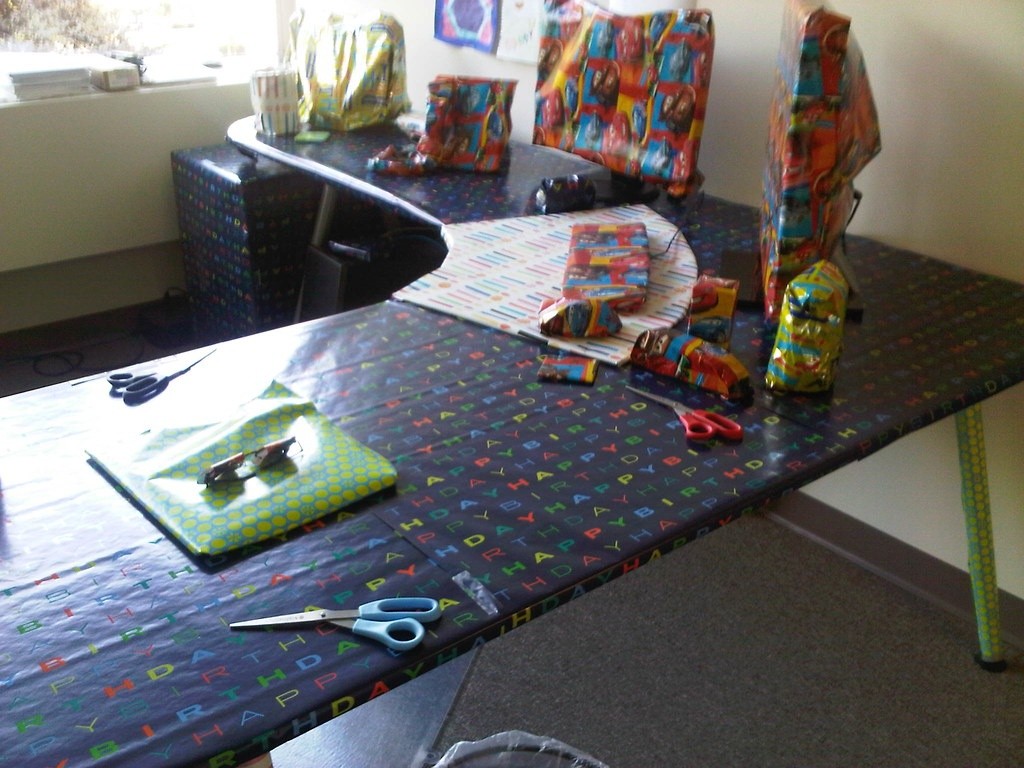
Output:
[251,69,300,136]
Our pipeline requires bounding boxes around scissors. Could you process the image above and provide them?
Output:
[228,596,442,653]
[106,347,218,400]
[624,384,743,441]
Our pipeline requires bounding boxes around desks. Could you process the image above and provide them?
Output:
[0,105,1024,768]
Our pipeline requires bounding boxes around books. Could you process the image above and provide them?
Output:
[0,52,94,102]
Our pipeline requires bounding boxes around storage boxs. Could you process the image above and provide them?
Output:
[169,145,387,349]
